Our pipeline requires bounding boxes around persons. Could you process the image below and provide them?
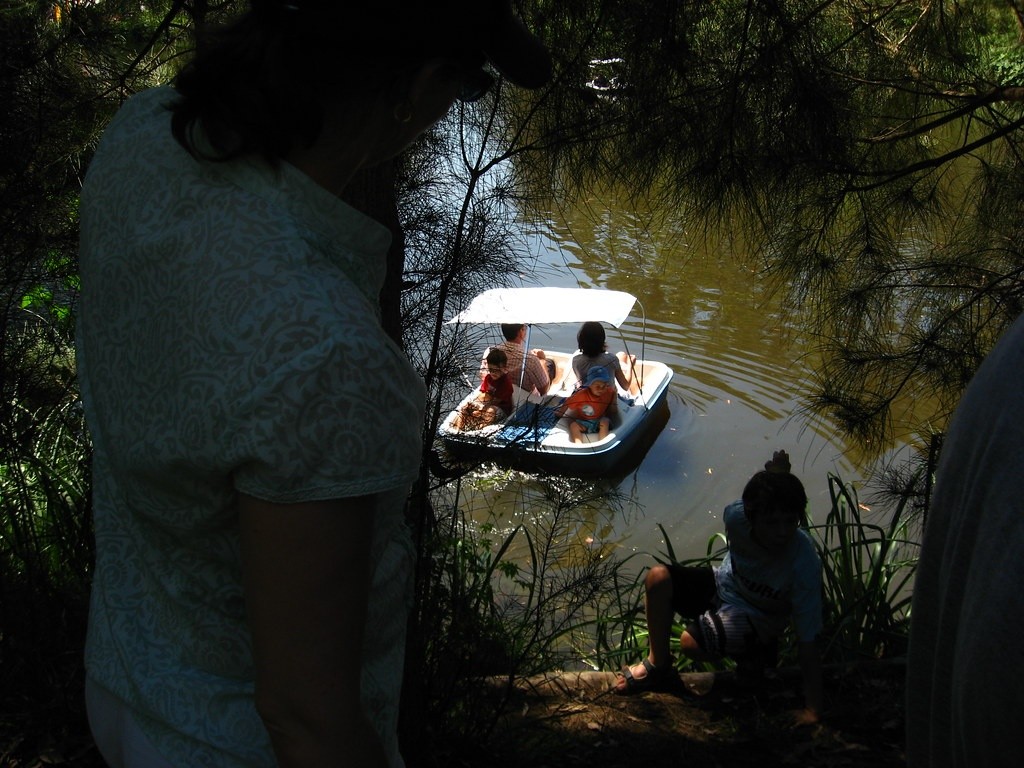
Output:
[611,472,829,726]
[71,0,556,768]
[459,321,636,444]
[903,315,1024,768]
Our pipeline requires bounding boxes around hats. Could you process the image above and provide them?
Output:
[587,366,611,386]
[244,0,555,87]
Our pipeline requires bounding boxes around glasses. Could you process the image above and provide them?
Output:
[443,54,495,103]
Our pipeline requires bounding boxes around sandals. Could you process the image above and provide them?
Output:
[615,659,678,698]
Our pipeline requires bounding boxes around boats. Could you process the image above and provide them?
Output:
[438,287,676,465]
